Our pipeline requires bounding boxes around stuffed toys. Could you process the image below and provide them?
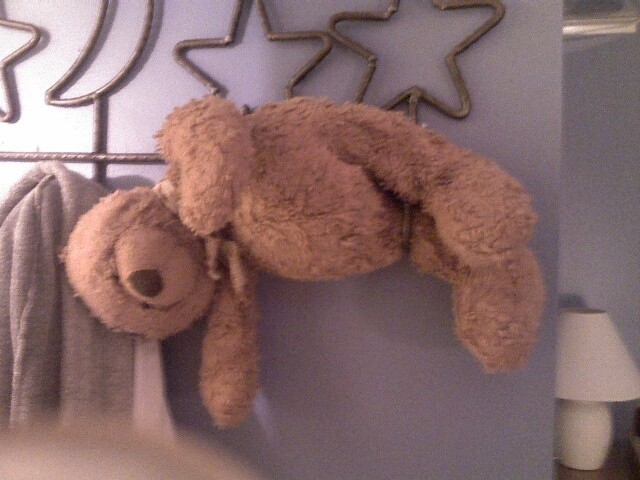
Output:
[55,93,546,434]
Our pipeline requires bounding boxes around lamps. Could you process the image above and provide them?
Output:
[553,308,640,471]
